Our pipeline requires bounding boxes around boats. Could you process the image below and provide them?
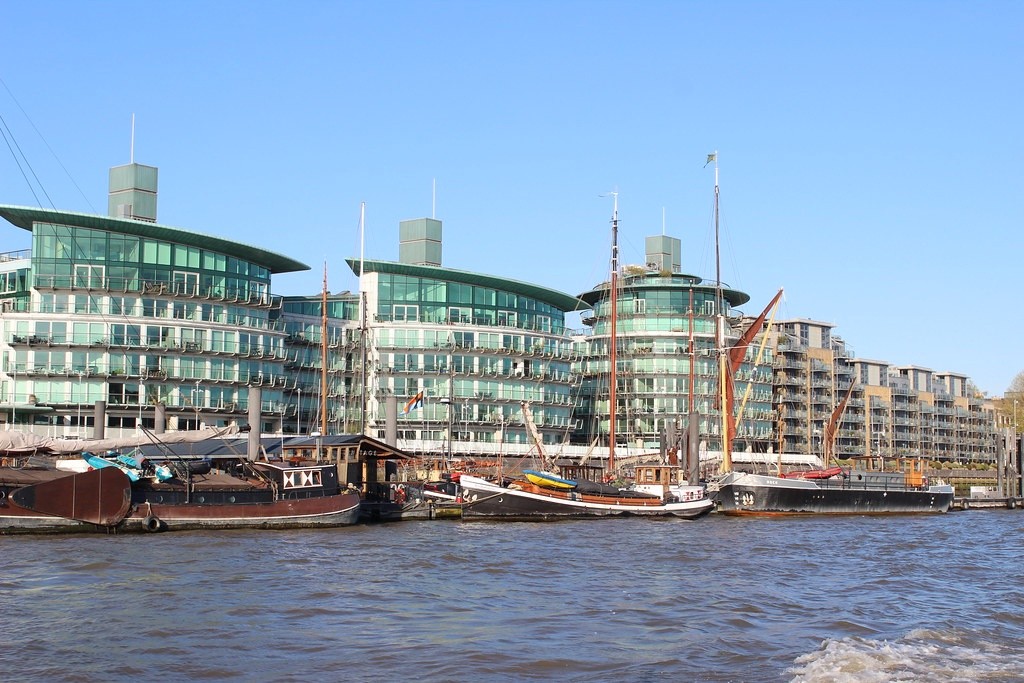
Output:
[459,465,715,523]
[718,456,954,517]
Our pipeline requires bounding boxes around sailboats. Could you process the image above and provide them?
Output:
[520,150,784,488]
[0,81,361,534]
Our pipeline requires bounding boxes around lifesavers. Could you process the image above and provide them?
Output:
[393,489,406,503]
[141,515,161,533]
